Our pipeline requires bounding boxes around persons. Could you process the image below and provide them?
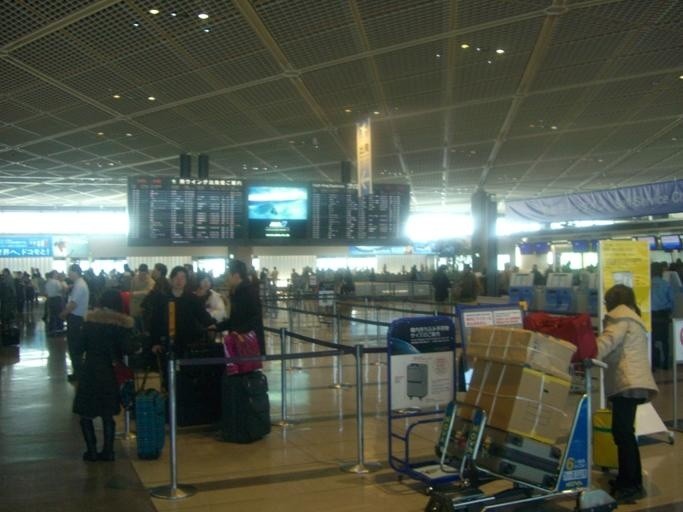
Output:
[356,264,478,303]
[71,290,135,462]
[650,258,683,371]
[593,283,661,502]
[0,268,68,332]
[531,261,572,286]
[62,259,266,381]
[261,265,352,291]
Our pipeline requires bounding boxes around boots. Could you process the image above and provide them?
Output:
[81,426,114,462]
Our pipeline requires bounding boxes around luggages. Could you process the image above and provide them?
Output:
[435,415,562,493]
[220,373,270,443]
[134,389,166,460]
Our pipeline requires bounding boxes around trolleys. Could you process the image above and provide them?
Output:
[425,359,617,512]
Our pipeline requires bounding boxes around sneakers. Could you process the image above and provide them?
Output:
[604,478,647,502]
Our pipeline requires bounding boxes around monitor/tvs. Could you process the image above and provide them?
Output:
[519,242,548,255]
[572,237,610,252]
[636,234,682,251]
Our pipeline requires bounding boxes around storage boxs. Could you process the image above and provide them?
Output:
[460,326,577,446]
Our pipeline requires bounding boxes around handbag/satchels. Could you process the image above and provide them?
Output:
[223,330,263,375]
[0,326,20,344]
[524,312,598,360]
